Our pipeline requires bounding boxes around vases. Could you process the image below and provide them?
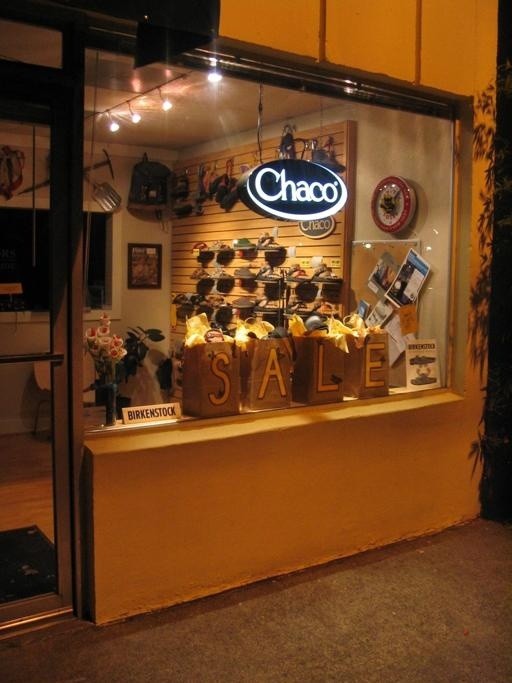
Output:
[100,382,118,426]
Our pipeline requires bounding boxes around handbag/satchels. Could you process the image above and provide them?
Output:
[182,329,240,416]
[343,314,390,400]
[240,316,293,409]
[293,316,343,406]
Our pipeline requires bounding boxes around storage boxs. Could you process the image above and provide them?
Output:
[0,310,51,355]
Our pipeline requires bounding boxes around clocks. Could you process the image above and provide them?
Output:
[370,175,418,235]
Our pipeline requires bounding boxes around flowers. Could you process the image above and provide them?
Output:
[83,311,166,385]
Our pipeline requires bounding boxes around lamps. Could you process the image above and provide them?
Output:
[105,86,173,133]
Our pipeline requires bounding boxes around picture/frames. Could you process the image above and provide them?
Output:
[127,242,162,290]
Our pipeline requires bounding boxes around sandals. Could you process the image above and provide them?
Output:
[312,301,339,318]
[286,265,313,281]
[286,300,312,316]
[254,295,284,313]
[257,264,282,281]
[257,236,284,249]
[313,265,343,283]
[170,240,233,308]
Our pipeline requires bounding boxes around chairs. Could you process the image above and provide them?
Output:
[32,358,52,439]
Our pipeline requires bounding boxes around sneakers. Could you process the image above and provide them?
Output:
[231,296,254,308]
[234,267,257,278]
[233,238,256,249]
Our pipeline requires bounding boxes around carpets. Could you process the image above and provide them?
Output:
[0,524,56,604]
